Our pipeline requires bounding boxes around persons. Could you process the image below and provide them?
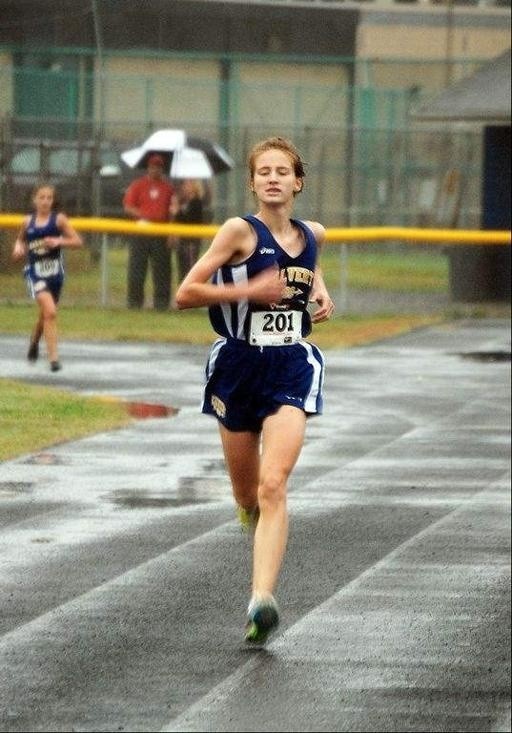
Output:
[120,154,171,309]
[176,136,336,643]
[169,180,213,286]
[10,181,85,372]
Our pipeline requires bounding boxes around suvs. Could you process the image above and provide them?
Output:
[0,129,156,254]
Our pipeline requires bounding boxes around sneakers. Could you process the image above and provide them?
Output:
[50,359,62,371]
[244,593,280,647]
[29,335,39,361]
[238,505,261,530]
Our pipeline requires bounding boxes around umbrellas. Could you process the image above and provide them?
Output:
[120,126,240,186]
